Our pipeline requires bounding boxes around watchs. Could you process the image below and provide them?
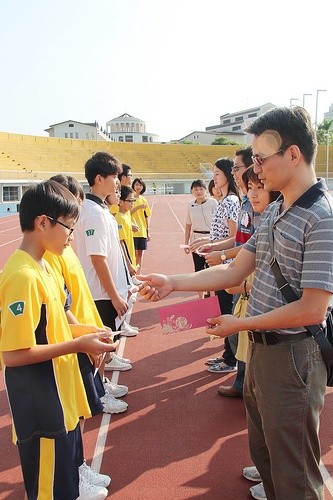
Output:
[220,250,226,261]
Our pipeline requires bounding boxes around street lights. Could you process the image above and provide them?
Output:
[314,89,328,137]
[302,93,312,108]
[290,98,299,106]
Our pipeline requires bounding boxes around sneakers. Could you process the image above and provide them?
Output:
[249,481,267,500]
[120,324,138,336]
[132,298,136,302]
[75,472,108,500]
[100,394,128,414]
[242,465,262,481]
[112,354,131,363]
[132,279,142,284]
[105,358,132,371]
[78,461,111,487]
[124,321,138,330]
[103,377,128,397]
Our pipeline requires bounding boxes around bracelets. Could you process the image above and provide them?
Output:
[241,279,250,300]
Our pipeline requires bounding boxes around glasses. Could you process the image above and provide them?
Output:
[127,174,132,177]
[251,143,301,166]
[37,215,75,235]
[124,199,136,202]
[232,164,246,172]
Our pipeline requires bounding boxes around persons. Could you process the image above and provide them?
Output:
[0,179,119,500]
[184,146,333,500]
[132,105,333,500]
[40,151,152,500]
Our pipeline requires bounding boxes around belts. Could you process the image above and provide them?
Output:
[247,329,312,345]
[193,230,210,234]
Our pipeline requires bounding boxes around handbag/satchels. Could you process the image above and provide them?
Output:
[326,313,333,387]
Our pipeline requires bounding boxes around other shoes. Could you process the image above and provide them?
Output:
[206,356,224,365]
[218,385,242,396]
[208,364,237,373]
